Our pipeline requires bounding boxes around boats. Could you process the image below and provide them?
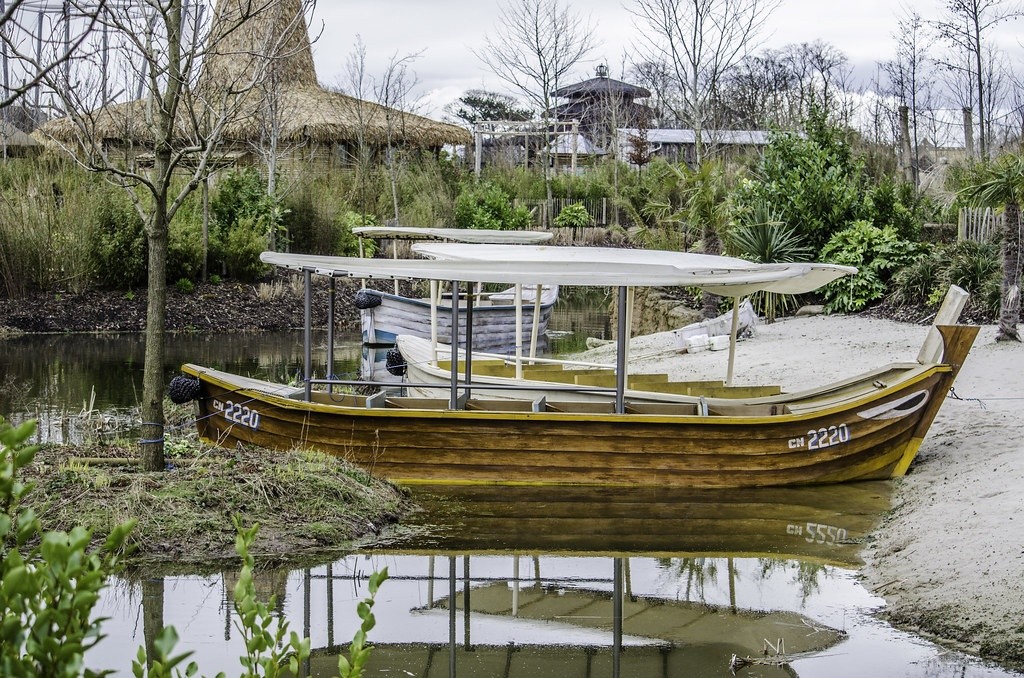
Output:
[276,481,897,678]
[352,226,559,346]
[397,241,858,404]
[169,250,980,489]
